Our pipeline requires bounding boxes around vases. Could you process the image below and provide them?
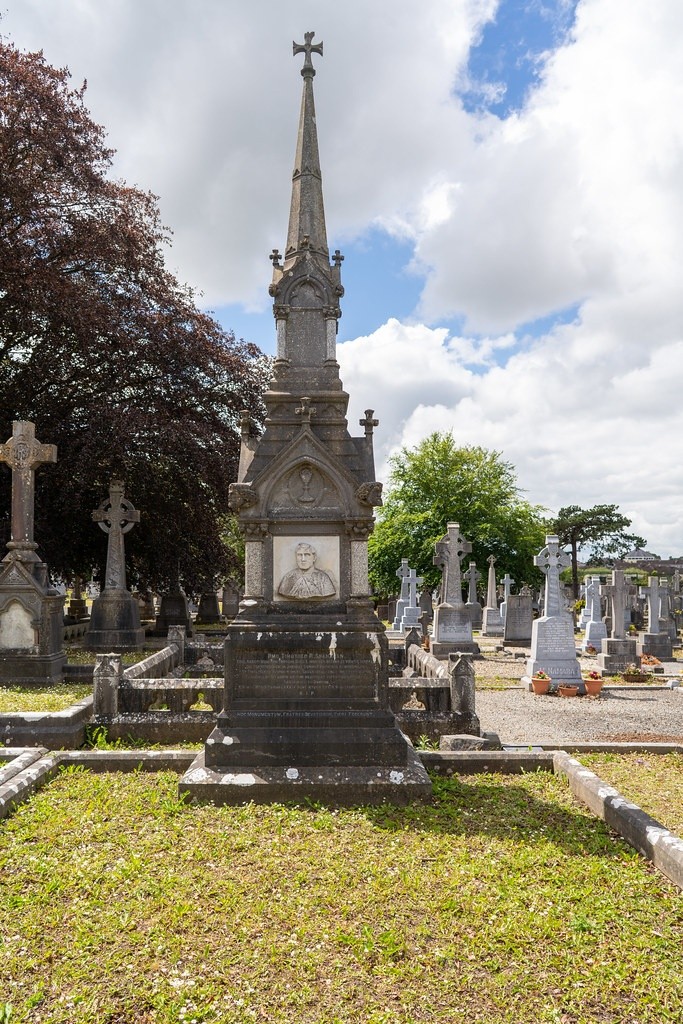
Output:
[558,685,580,697]
[584,679,604,696]
[531,677,551,695]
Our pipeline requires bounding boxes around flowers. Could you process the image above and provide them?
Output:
[536,671,547,678]
[589,671,602,680]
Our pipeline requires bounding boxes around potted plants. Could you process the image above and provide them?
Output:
[622,663,652,683]
[628,624,637,636]
